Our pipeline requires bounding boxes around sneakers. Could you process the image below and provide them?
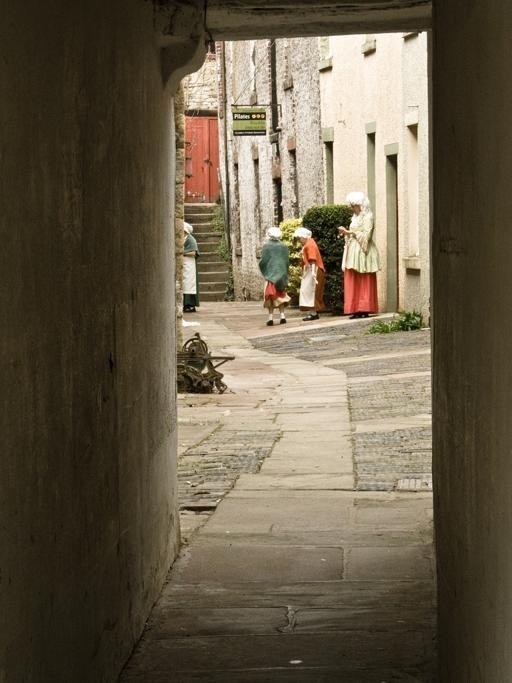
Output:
[350,313,368,318]
[267,320,273,326]
[183,306,195,312]
[303,314,319,321]
[280,319,286,324]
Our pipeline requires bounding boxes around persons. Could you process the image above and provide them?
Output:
[293,228,327,322]
[183,219,202,312]
[336,191,382,321]
[258,225,292,327]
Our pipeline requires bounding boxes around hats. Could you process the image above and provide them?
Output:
[183,222,193,234]
[268,227,282,240]
[294,228,311,238]
[347,192,370,206]
